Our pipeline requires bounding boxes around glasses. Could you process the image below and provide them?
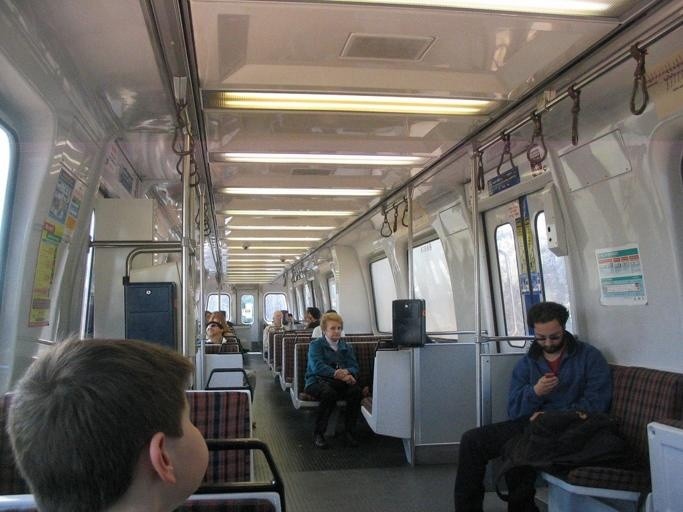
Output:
[535,333,563,341]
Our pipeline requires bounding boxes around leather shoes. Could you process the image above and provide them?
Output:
[338,429,359,447]
[312,432,328,447]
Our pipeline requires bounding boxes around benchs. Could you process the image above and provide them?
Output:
[536,362,683,511]
[1,387,283,510]
[262,323,460,468]
[195,324,246,389]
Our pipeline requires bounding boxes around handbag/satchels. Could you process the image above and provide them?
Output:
[505,410,612,469]
[317,362,361,387]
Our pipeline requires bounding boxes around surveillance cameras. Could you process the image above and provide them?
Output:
[244,245,247,250]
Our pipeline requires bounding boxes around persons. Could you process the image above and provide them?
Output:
[204,310,249,354]
[304,306,321,330]
[280,309,295,327]
[311,309,346,338]
[204,321,258,429]
[302,311,363,450]
[5,331,211,512]
[208,311,231,333]
[261,310,286,359]
[452,300,613,512]
[287,313,300,324]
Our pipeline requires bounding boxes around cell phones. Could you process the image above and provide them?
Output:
[545,373,555,378]
